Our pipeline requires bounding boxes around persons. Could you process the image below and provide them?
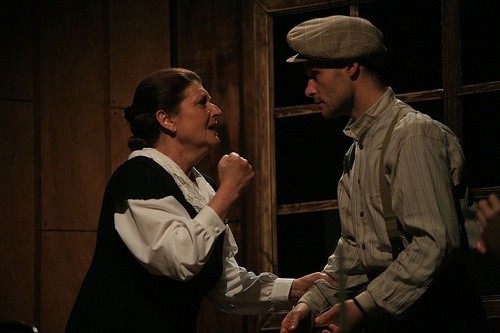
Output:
[279,14,490,332]
[64,67,337,332]
[469,190,500,257]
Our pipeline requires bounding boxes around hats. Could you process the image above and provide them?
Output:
[286,15,384,63]
